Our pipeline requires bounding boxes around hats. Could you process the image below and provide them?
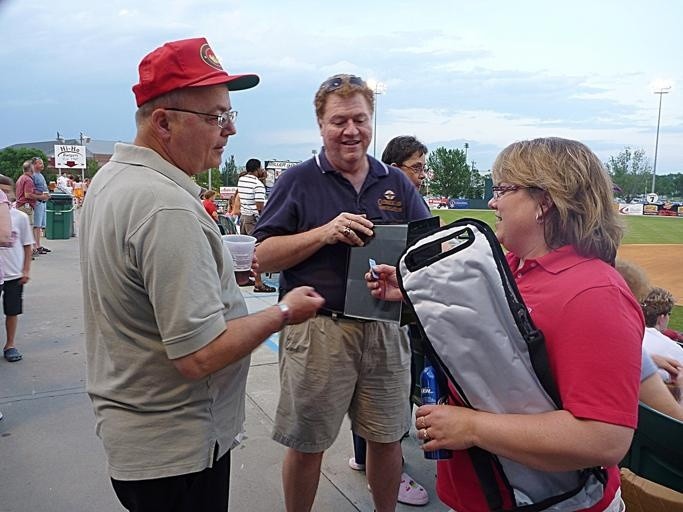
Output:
[132,37,258,105]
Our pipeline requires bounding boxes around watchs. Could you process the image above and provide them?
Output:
[272,301,292,328]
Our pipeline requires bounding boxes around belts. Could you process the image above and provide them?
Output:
[38,199,46,204]
[316,306,385,321]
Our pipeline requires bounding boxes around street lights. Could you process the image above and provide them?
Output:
[652,86,671,194]
[56,129,66,175]
[79,131,90,180]
[368,82,385,158]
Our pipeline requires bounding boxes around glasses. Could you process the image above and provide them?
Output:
[164,105,238,127]
[399,162,432,173]
[320,73,367,92]
[489,182,541,196]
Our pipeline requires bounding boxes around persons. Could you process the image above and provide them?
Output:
[32,157,52,256]
[228,159,276,293]
[612,260,682,422]
[0,174,36,362]
[200,189,222,224]
[365,136,645,512]
[78,34,327,512]
[0,188,12,323]
[49,173,91,207]
[257,72,433,512]
[381,134,433,404]
[15,160,48,258]
[349,395,428,505]
[640,286,683,386]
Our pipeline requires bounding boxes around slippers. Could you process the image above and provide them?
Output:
[4,346,23,362]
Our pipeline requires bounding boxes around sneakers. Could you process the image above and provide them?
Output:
[368,472,429,505]
[37,246,47,254]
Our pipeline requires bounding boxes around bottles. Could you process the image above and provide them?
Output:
[419,355,454,460]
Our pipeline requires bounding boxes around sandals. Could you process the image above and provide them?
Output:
[254,284,277,293]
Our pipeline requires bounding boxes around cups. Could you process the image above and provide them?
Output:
[221,235,257,272]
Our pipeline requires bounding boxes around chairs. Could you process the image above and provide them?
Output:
[630,401,683,492]
[618,466,683,512]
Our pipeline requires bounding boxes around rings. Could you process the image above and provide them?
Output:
[342,220,352,236]
[418,415,430,440]
